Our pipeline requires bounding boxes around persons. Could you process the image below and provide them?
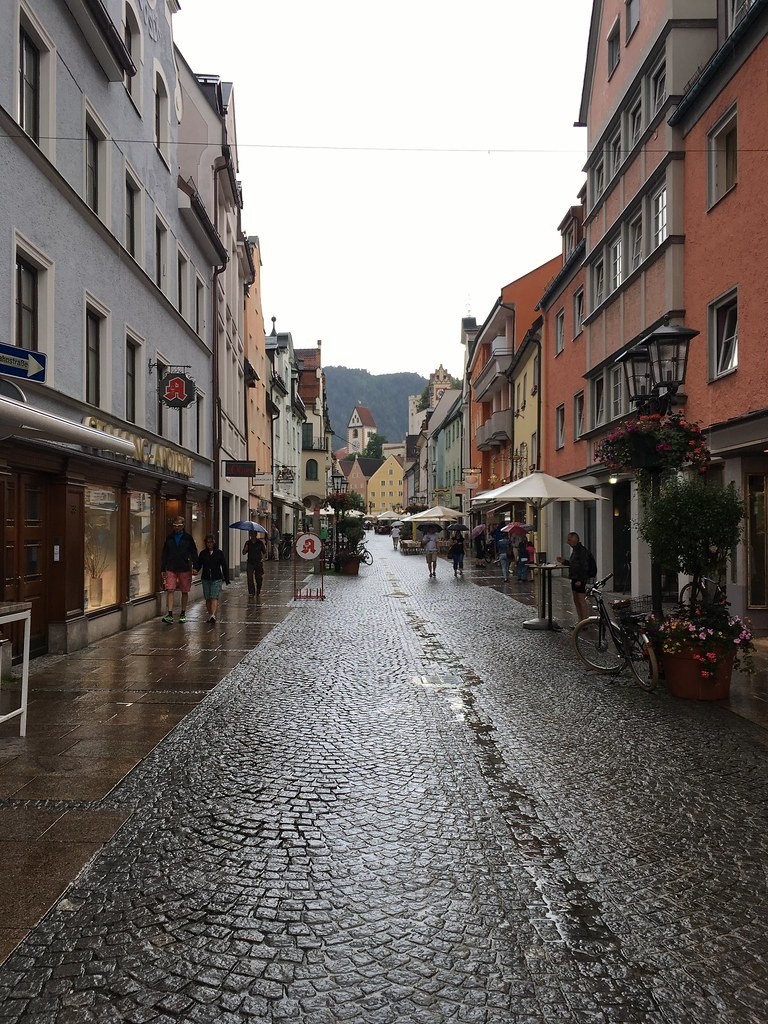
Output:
[476,535,539,582]
[320,527,328,543]
[193,534,231,623]
[421,527,440,577]
[243,529,267,599]
[366,522,371,532]
[447,531,467,576]
[161,516,198,624]
[297,530,305,541]
[388,526,402,550]
[557,532,597,628]
[268,524,281,562]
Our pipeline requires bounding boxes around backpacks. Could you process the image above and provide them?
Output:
[585,548,598,577]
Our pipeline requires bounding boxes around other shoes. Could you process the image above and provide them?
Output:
[460,572,464,575]
[475,564,481,567]
[481,565,486,568]
[209,616,217,624]
[433,573,437,576]
[455,573,458,576]
[570,621,588,630]
[430,574,433,578]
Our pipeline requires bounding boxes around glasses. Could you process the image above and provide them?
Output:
[173,524,183,527]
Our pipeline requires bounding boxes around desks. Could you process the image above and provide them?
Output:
[0,599,32,735]
[524,563,571,632]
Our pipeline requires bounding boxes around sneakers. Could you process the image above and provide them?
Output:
[178,615,186,623]
[162,615,174,624]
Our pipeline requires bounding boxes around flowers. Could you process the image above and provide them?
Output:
[593,413,712,479]
[637,594,758,678]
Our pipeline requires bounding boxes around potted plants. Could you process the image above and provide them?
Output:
[335,549,361,574]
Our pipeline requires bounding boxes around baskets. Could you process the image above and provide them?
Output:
[628,594,654,614]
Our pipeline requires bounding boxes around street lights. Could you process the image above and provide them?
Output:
[616,311,701,621]
[331,469,345,572]
[341,479,349,550]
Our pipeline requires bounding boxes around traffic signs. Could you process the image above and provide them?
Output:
[0,342,48,385]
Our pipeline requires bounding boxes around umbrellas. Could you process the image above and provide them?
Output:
[501,522,536,534]
[489,523,509,540]
[468,471,610,631]
[229,521,268,540]
[445,523,469,531]
[470,524,486,541]
[416,523,443,533]
[339,509,413,531]
[135,509,150,533]
[391,521,404,527]
[400,505,467,528]
[305,507,338,515]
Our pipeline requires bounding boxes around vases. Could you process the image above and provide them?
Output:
[89,576,102,606]
[669,644,730,698]
[637,443,667,474]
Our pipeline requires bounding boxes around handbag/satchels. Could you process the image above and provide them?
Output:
[447,551,453,560]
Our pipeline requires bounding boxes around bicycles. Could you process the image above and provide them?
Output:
[680,571,726,609]
[351,539,374,565]
[484,539,497,563]
[570,573,660,692]
[276,539,293,560]
